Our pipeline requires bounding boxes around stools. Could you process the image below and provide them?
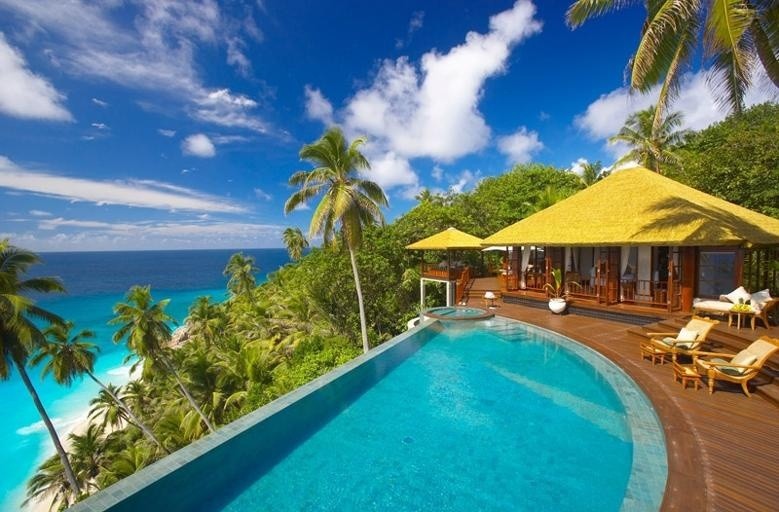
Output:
[640,345,665,365]
[672,365,701,391]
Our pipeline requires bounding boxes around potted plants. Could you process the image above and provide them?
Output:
[541,266,584,314]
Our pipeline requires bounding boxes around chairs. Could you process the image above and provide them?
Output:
[647,314,721,365]
[694,335,779,398]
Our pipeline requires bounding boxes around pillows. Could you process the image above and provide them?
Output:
[730,350,756,374]
[676,329,697,348]
[749,289,772,311]
[726,286,750,304]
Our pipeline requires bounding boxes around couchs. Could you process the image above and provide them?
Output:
[692,293,779,330]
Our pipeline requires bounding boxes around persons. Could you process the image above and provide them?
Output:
[438,254,449,269]
[499,251,507,269]
[623,265,635,282]
[657,257,667,289]
